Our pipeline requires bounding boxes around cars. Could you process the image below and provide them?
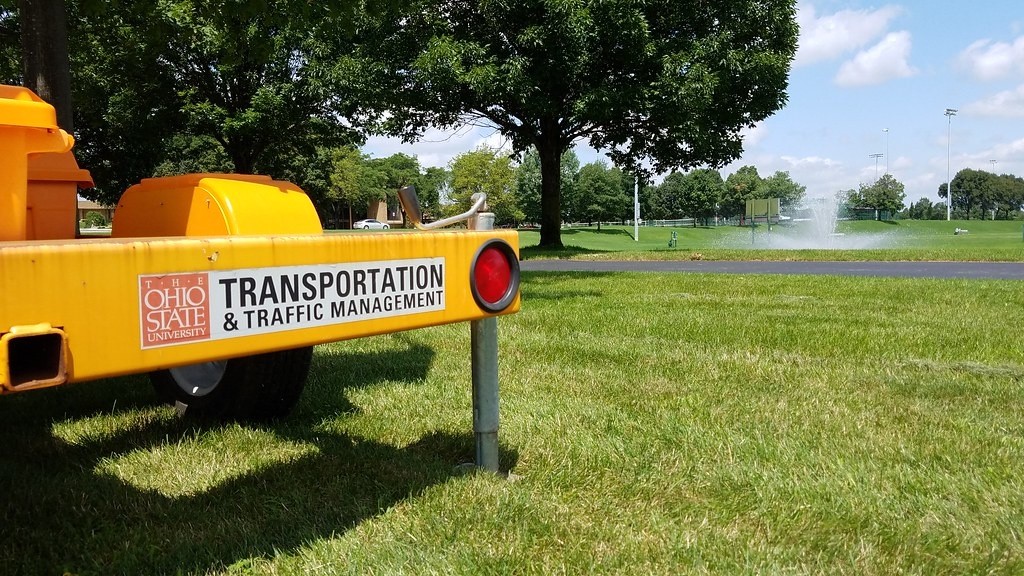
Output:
[353,219,390,230]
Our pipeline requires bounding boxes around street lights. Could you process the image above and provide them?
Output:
[943,108,959,221]
[881,128,889,175]
[869,152,884,221]
